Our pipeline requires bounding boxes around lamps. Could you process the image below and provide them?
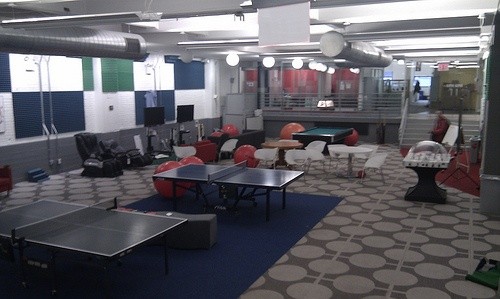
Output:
[180,48,192,63]
[292,59,303,69]
[308,62,335,75]
[262,56,275,68]
[226,53,239,66]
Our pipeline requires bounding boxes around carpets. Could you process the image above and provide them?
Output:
[400,147,480,197]
[0,185,345,299]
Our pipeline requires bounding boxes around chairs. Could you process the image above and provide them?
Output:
[172,140,389,186]
[78,131,154,177]
[0,165,13,197]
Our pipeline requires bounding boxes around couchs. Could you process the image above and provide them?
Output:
[209,130,265,158]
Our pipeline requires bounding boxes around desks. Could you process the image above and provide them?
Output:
[0,199,189,299]
[152,163,304,221]
[291,126,353,154]
[261,141,304,168]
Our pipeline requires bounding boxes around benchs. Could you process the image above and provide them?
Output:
[136,211,218,249]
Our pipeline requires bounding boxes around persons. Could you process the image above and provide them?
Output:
[433,110,449,132]
